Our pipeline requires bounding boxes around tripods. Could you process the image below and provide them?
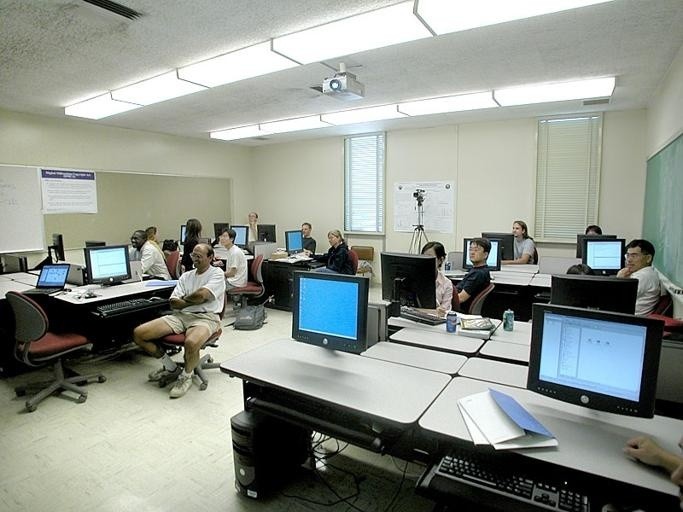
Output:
[407,208,430,254]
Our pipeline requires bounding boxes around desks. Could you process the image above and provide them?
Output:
[0,259,180,365]
[180,245,324,313]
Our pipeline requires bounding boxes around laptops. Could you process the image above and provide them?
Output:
[21,263,70,295]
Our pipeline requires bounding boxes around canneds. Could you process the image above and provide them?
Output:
[446,312,457,332]
[503,309,514,331]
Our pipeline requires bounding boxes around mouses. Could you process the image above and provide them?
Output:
[148,296,161,301]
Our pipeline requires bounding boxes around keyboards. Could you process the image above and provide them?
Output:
[424,446,590,511]
[249,390,386,447]
[96,300,133,314]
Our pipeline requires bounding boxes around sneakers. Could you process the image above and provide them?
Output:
[149,367,179,381]
[169,375,192,398]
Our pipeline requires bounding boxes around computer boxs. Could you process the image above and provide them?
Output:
[230,409,314,504]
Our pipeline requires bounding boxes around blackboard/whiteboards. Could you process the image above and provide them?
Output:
[45,168,232,252]
[0,165,45,254]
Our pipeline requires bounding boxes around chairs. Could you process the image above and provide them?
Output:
[347,250,358,273]
[164,252,181,280]
[157,291,227,390]
[532,248,540,266]
[5,290,106,412]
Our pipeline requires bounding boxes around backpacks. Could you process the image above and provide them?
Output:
[233,305,264,330]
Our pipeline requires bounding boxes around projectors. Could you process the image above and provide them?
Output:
[321,72,368,103]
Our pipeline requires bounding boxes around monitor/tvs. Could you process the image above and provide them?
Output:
[576,233,617,257]
[179,224,188,243]
[213,222,228,237]
[462,237,502,272]
[284,229,304,255]
[229,225,248,248]
[481,231,514,261]
[83,244,132,287]
[379,249,437,317]
[291,269,368,358]
[549,274,638,316]
[46,232,66,261]
[581,237,625,275]
[524,301,666,421]
[256,223,276,243]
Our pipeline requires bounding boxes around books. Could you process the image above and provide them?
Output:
[457,317,495,339]
[459,318,495,330]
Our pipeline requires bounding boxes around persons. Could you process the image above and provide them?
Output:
[180,219,203,272]
[243,212,259,246]
[615,238,661,315]
[567,263,595,276]
[145,226,161,244]
[622,431,682,499]
[218,228,249,320]
[452,237,491,317]
[412,241,456,319]
[500,220,536,265]
[302,229,356,277]
[301,222,316,253]
[131,229,173,280]
[132,244,226,398]
[584,225,604,236]
